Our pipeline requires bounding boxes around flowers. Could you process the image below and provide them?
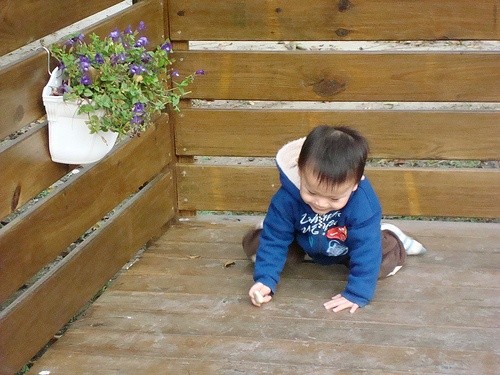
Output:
[49,18,207,149]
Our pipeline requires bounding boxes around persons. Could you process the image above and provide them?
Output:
[248,123,427,315]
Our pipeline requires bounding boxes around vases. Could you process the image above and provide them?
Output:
[41,64,135,165]
